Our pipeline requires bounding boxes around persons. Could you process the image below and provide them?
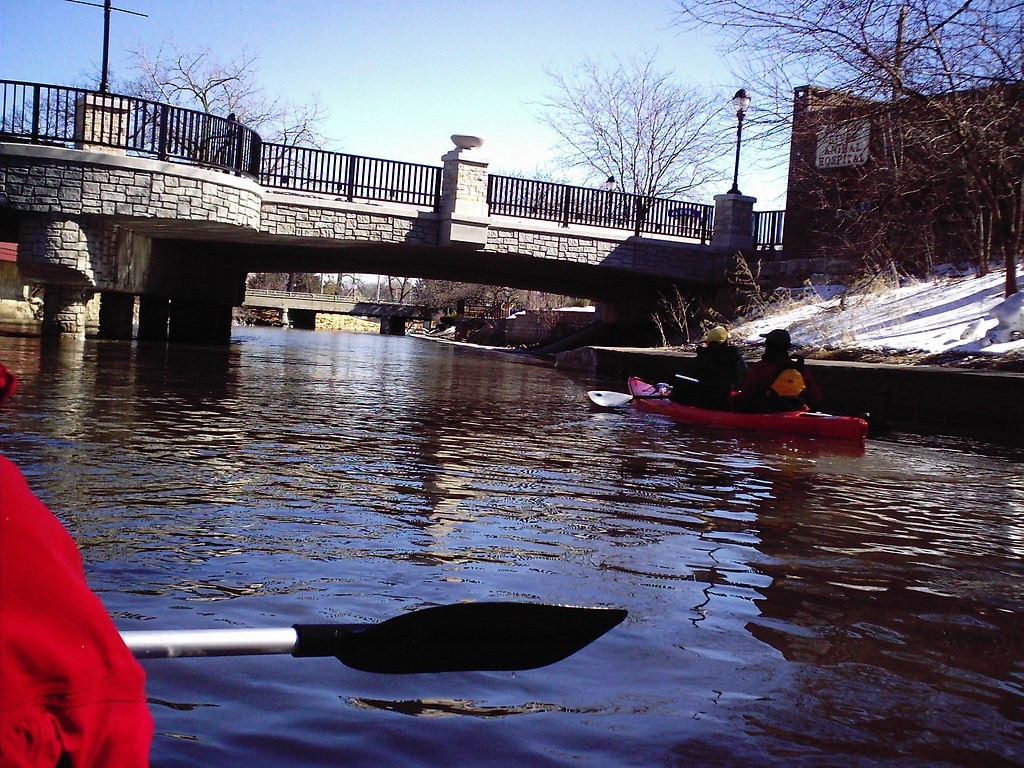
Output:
[739,329,823,414]
[685,325,747,412]
[0,360,156,768]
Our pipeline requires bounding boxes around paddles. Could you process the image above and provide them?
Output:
[117,601,630,676]
[586,391,670,408]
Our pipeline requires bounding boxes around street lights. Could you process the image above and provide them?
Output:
[726,88,752,195]
[605,175,617,227]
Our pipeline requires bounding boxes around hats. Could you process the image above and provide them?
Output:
[707,326,728,344]
[760,329,792,350]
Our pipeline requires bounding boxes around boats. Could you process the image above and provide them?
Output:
[627,376,870,441]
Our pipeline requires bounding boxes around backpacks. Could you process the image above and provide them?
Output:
[771,353,805,411]
